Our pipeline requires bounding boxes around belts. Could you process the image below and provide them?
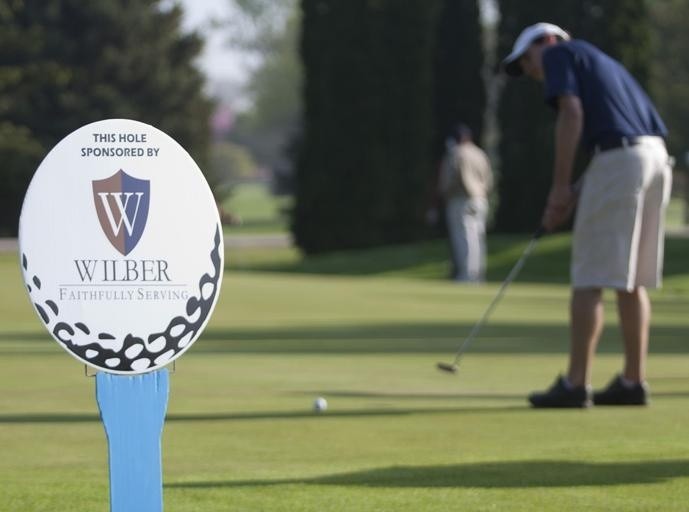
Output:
[588,134,672,155]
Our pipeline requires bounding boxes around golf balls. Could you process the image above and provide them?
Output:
[313,397,326,409]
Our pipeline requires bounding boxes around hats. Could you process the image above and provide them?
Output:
[498,19,574,78]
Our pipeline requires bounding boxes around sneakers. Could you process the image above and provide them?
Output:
[526,373,591,409]
[592,373,651,406]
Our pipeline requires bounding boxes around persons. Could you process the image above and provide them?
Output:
[502,22,673,410]
[436,120,492,281]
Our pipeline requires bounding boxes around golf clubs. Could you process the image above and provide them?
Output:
[437,227,546,372]
